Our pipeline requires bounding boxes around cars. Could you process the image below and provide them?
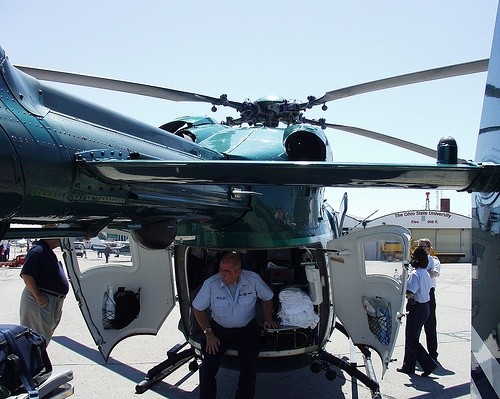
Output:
[73,238,131,258]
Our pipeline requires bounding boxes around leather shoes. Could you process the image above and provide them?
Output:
[423,363,438,377]
[398,365,416,376]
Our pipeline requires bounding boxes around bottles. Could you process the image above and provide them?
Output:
[394,268,399,281]
[106,286,116,319]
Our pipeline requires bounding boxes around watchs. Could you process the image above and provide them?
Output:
[204,328,211,333]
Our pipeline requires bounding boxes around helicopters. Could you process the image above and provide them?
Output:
[0,46,490,399]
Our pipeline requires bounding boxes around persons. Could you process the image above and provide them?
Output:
[191,253,280,399]
[105,244,110,262]
[400,238,441,362]
[396,247,438,377]
[19,223,70,350]
[1,240,11,259]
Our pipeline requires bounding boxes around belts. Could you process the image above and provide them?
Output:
[39,288,67,299]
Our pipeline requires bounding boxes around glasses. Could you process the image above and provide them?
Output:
[416,244,429,251]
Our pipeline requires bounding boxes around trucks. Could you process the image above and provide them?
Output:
[380,238,436,262]
